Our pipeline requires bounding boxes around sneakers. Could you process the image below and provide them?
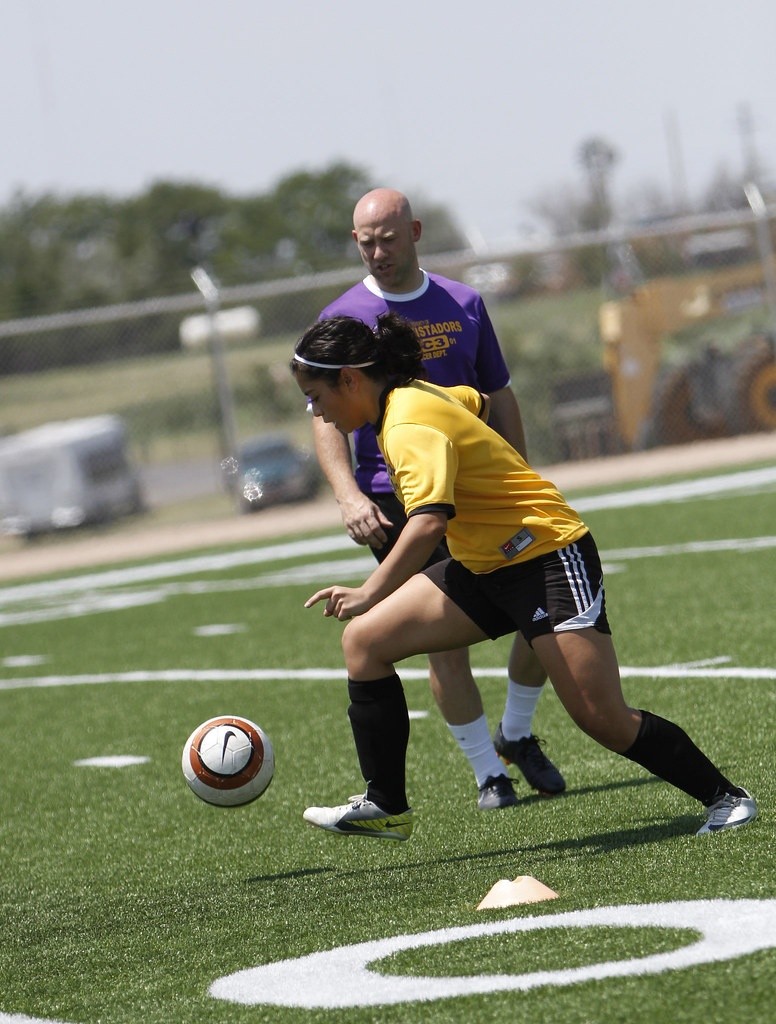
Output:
[477,773,519,810]
[302,780,413,841]
[696,786,757,836]
[493,721,566,796]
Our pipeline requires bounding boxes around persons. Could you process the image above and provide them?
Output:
[304,189,566,810]
[287,310,763,841]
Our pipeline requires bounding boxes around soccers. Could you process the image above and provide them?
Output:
[181,715,276,808]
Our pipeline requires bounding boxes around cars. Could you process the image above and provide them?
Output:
[240,439,317,511]
[552,375,613,459]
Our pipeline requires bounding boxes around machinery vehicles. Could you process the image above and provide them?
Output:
[599,257,776,450]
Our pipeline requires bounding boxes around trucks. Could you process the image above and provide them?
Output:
[0,414,142,537]
